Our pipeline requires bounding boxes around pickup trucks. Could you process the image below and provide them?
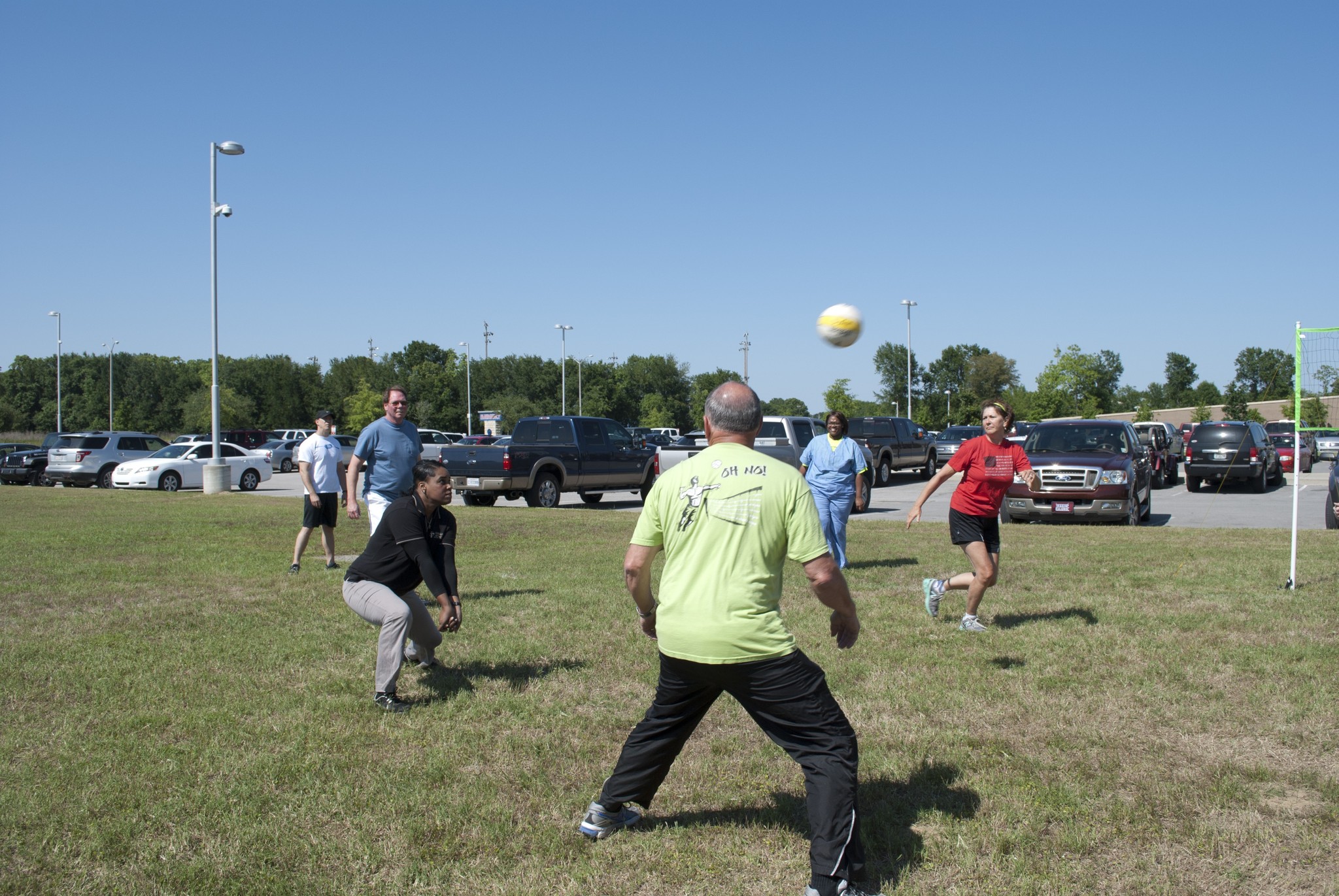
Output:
[439,415,656,509]
[652,415,876,514]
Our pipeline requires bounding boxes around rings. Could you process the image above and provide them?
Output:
[1029,474,1033,478]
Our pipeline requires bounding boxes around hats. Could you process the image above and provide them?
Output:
[316,410,336,420]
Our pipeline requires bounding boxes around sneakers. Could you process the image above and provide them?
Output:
[374,686,412,713]
[403,646,440,668]
[578,800,641,839]
[922,577,944,618]
[958,616,989,631]
[803,879,871,896]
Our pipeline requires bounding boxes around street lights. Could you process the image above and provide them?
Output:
[566,355,592,416]
[202,140,244,494]
[554,325,573,416]
[483,321,493,361]
[607,352,618,370]
[367,338,378,359]
[739,330,751,386]
[797,406,802,415]
[944,389,950,427]
[891,401,899,420]
[459,342,472,436]
[308,355,318,365]
[901,299,919,421]
[102,340,120,432]
[48,310,63,434]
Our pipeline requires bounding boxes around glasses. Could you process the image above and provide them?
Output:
[386,401,407,407]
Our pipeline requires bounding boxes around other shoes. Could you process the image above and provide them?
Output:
[325,563,342,571]
[287,564,300,575]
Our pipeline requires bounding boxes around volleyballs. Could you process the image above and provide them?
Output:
[816,302,861,347]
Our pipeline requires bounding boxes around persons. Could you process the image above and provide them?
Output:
[797,410,869,570]
[579,382,873,896]
[342,459,462,716]
[346,385,432,606]
[906,401,1042,633]
[287,409,348,575]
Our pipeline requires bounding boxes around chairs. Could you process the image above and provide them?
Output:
[1048,432,1120,453]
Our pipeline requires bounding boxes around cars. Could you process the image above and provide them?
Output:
[933,416,1201,488]
[415,429,512,464]
[536,433,625,442]
[110,428,369,492]
[624,427,713,446]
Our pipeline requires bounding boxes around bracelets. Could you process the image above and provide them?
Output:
[452,601,462,607]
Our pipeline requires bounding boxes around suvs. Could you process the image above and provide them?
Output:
[1001,420,1155,528]
[0,432,171,489]
[839,415,938,483]
[1184,419,1339,530]
